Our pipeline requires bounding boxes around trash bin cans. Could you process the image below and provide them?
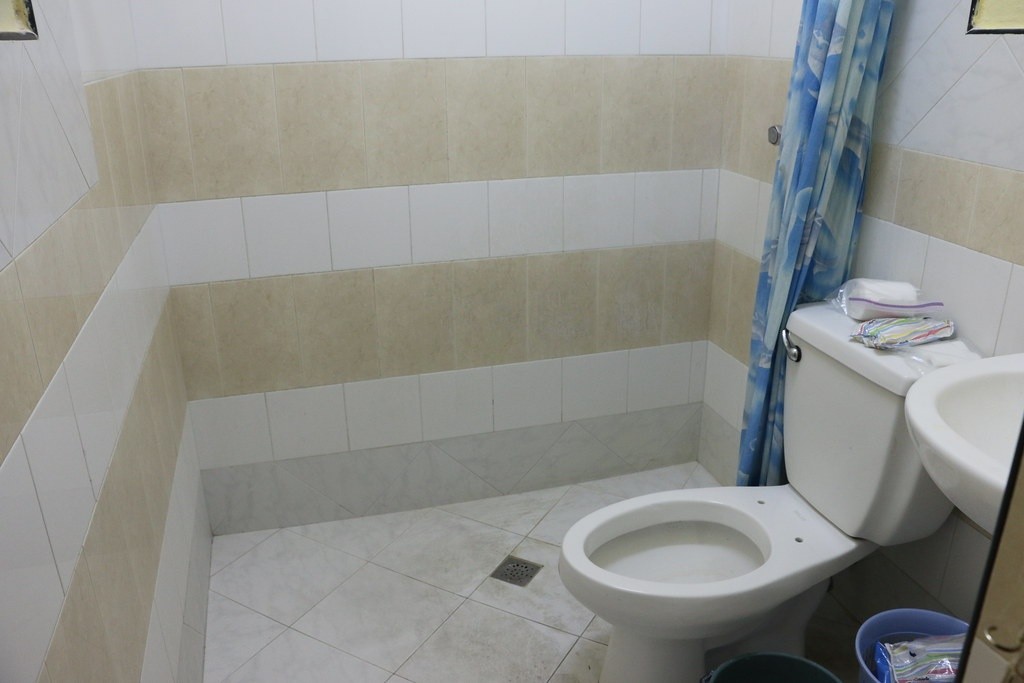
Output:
[854,609,969,683]
[710,652,841,683]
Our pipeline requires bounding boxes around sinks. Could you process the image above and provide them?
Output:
[903,351,1024,539]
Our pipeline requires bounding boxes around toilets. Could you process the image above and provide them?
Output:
[556,297,980,683]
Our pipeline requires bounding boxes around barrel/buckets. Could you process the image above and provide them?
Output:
[703,652,842,683]
[855,608,970,683]
